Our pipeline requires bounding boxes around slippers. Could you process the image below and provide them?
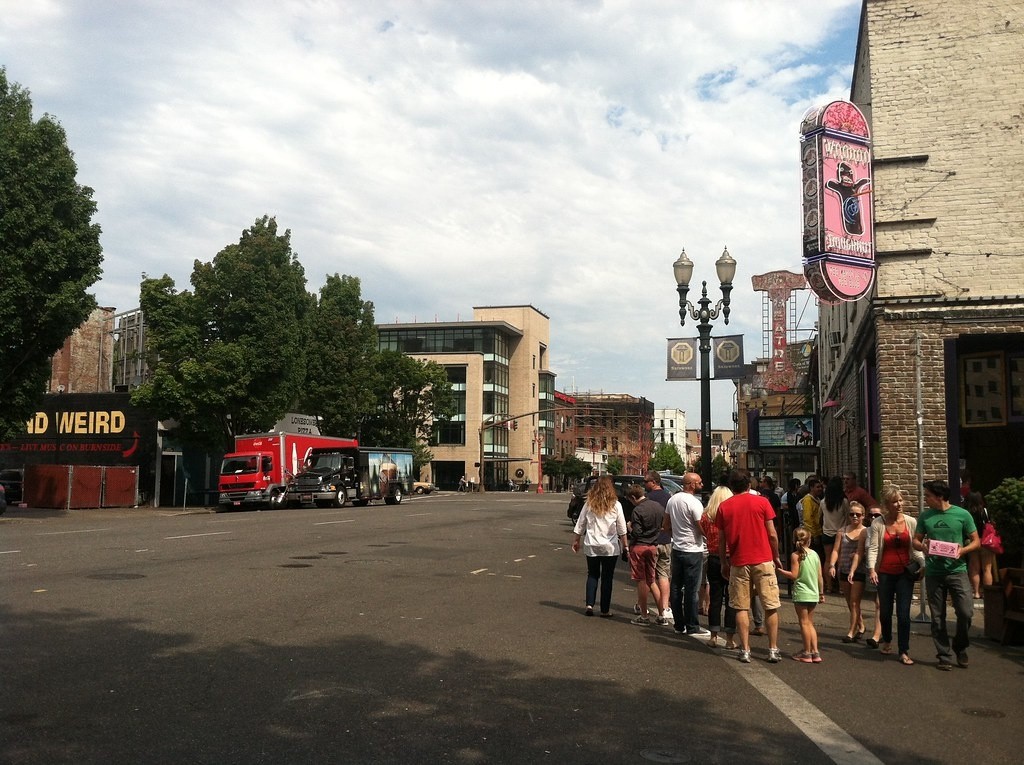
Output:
[899,655,913,665]
[881,646,892,655]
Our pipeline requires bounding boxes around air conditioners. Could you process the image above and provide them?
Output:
[830,331,841,348]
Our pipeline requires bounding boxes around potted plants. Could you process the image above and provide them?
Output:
[983,476,1024,586]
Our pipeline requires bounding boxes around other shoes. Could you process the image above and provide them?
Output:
[672,625,686,634]
[665,607,674,619]
[706,638,718,647]
[599,612,614,617]
[866,635,884,649]
[633,603,642,615]
[842,636,857,643]
[584,605,594,617]
[688,627,711,636]
[855,627,866,639]
[748,627,764,635]
[725,641,738,649]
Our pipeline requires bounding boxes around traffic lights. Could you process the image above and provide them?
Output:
[502,420,507,430]
[513,420,518,431]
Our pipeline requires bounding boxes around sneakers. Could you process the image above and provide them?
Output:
[631,616,650,627]
[654,616,669,626]
[952,648,969,668]
[768,648,782,662]
[791,649,822,663]
[739,646,752,663]
[938,659,952,671]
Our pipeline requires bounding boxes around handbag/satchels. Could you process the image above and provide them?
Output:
[904,560,920,581]
[980,524,1005,554]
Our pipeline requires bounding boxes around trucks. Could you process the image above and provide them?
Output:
[218,431,359,513]
[286,446,414,510]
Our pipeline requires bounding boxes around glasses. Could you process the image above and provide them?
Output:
[848,512,862,518]
[867,513,882,519]
[895,535,901,549]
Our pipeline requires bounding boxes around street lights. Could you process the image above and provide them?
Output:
[533,429,546,494]
[588,440,600,475]
[674,244,736,499]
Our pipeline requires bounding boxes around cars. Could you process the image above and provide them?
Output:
[402,480,438,495]
[566,473,718,525]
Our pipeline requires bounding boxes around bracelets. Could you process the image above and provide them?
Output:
[625,546,628,547]
[828,565,835,569]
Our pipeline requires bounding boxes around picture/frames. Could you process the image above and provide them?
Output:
[959,350,1007,428]
[1005,352,1024,423]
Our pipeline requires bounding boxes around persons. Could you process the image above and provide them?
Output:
[961,489,995,599]
[828,501,885,649]
[571,475,630,618]
[749,472,877,596]
[626,468,825,663]
[865,479,981,672]
[457,475,468,492]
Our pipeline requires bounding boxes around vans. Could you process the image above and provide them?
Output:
[582,474,683,527]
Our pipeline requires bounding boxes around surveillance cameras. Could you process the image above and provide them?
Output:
[833,406,850,420]
